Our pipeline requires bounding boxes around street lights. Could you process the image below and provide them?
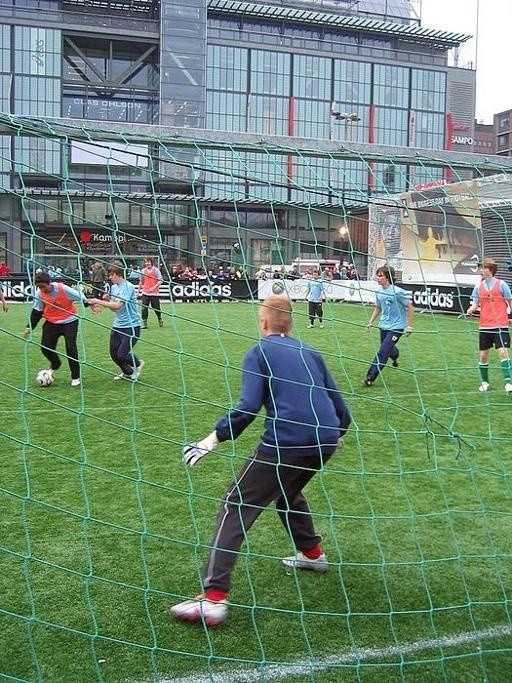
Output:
[334,111,361,273]
[105,214,117,263]
[340,226,346,272]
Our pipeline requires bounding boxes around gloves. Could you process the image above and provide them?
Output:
[182,430,220,468]
[365,321,373,331]
[406,326,413,336]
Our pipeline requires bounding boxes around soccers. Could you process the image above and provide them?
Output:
[37,369,54,387]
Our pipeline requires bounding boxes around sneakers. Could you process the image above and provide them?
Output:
[320,322,324,328]
[70,378,80,386]
[158,320,163,327]
[131,359,145,380]
[392,359,399,368]
[307,324,314,329]
[170,593,229,628]
[141,325,147,329]
[48,368,57,375]
[479,381,489,392]
[505,383,512,393]
[361,379,373,386]
[283,551,330,574]
[114,373,132,381]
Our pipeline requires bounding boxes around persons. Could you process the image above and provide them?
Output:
[306,270,325,328]
[23,272,102,386]
[88,260,145,381]
[380,208,404,267]
[362,265,414,387]
[39,261,144,297]
[465,257,511,394]
[138,258,163,329]
[1,263,11,313]
[255,266,361,303]
[168,295,351,626]
[160,263,249,303]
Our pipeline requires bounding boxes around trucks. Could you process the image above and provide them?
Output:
[258,259,354,280]
